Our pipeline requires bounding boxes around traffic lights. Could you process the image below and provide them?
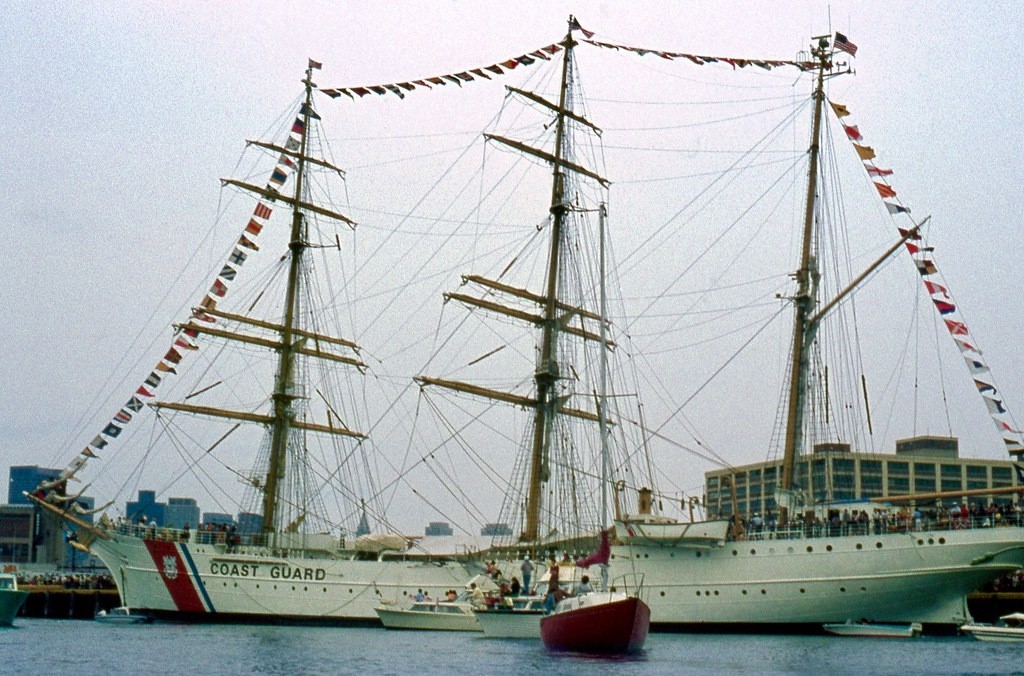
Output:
[540,573,651,654]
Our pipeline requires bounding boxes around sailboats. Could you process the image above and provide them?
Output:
[13,10,1024,636]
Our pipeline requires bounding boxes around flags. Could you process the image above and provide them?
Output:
[834,32,857,55]
[574,18,594,39]
[583,40,832,70]
[831,102,1024,457]
[321,41,571,99]
[309,59,322,69]
[36,106,321,498]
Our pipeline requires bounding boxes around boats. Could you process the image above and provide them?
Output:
[610,516,731,550]
[823,619,923,637]
[95,607,148,624]
[0,574,25,626]
[373,600,484,632]
[965,592,1024,623]
[961,612,1024,644]
[471,565,601,637]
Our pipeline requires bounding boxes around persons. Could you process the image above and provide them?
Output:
[466,583,484,609]
[574,575,591,595]
[445,589,459,601]
[731,502,1024,539]
[16,573,116,589]
[992,572,1024,592]
[545,587,572,611]
[399,589,429,603]
[197,521,236,544]
[117,514,190,541]
[488,556,534,595]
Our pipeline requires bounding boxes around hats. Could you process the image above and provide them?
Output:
[524,556,529,560]
